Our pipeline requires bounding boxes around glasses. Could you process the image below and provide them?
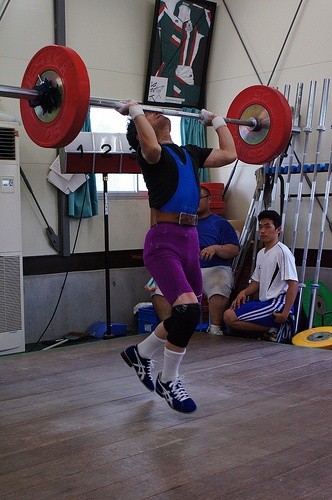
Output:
[200,194,210,200]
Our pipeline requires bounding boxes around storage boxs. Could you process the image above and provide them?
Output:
[137,307,159,335]
[88,322,128,339]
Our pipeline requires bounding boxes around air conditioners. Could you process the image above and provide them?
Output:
[0,120,25,356]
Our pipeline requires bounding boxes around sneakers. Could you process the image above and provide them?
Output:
[120,344,155,392]
[155,370,197,414]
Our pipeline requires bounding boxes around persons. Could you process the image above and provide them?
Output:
[150,186,241,334]
[224,209,299,338]
[115,98,237,413]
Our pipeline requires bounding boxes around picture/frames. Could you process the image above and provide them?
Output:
[143,0,218,110]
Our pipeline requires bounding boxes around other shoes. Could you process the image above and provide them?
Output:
[208,328,223,336]
[267,327,278,342]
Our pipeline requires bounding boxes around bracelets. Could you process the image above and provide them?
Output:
[129,105,145,120]
[212,117,227,131]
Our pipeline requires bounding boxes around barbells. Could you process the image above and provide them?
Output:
[0,44,302,167]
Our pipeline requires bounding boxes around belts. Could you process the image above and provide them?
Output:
[149,209,199,229]
